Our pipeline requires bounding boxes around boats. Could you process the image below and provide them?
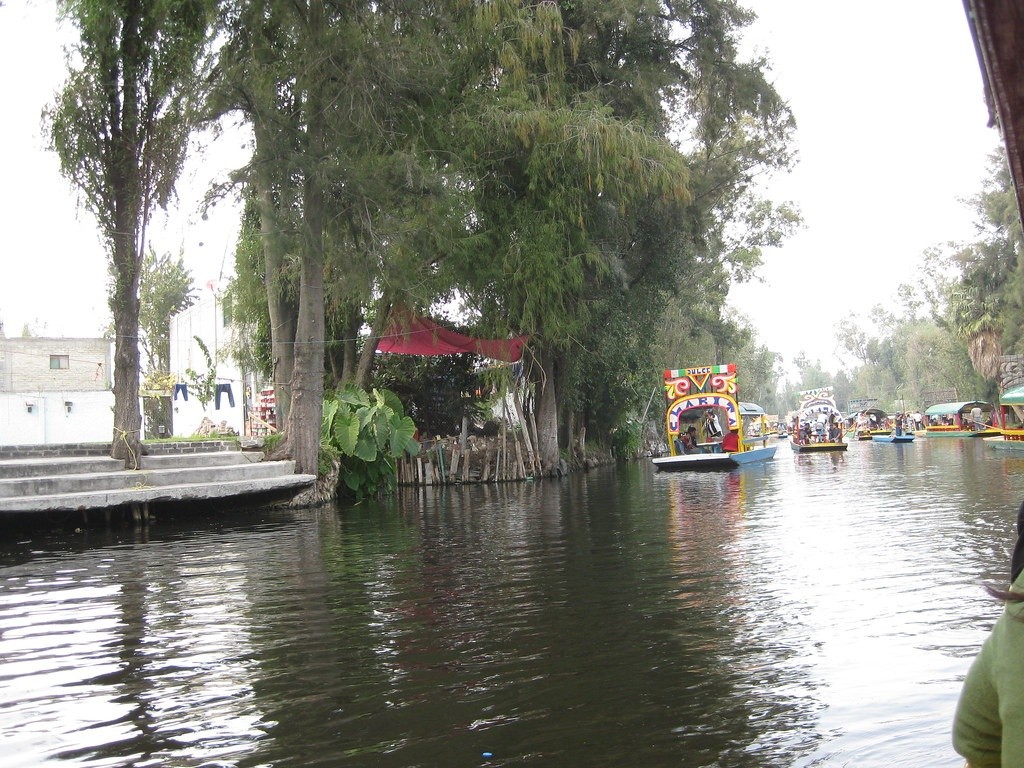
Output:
[651,365,778,466]
[737,386,1024,452]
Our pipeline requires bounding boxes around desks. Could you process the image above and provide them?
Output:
[809,434,827,443]
[696,441,723,454]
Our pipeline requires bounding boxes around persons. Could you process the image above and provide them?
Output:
[951,501,1024,768]
[742,404,991,445]
[671,409,739,456]
